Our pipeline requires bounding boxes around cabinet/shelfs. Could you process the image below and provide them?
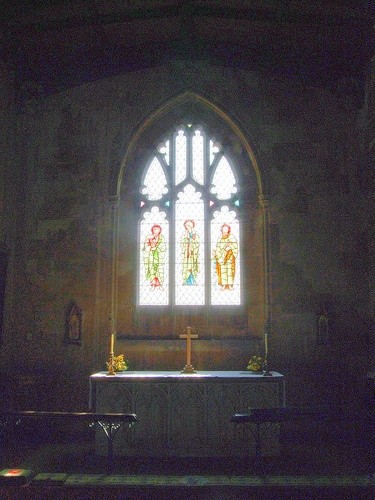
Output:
[90,371,284,457]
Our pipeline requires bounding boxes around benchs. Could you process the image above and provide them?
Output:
[0,411,138,475]
[231,409,357,467]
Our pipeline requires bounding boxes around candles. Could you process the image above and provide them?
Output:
[265,335,268,357]
[110,333,115,353]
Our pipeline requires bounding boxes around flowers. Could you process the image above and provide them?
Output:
[106,355,126,372]
[247,357,265,372]
